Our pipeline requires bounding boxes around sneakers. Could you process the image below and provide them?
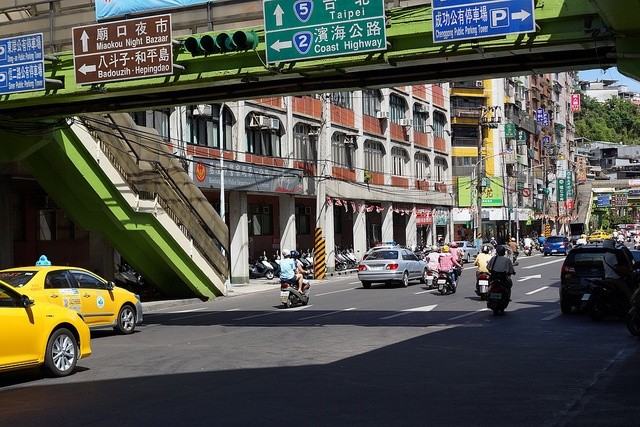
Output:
[298,290,303,295]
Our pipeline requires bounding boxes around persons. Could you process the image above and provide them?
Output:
[449,242,464,264]
[490,236,499,245]
[290,250,310,292]
[426,244,441,271]
[524,234,534,251]
[475,246,493,279]
[538,233,547,247]
[576,234,588,246]
[612,229,632,243]
[280,250,304,296]
[510,237,519,260]
[436,245,459,287]
[475,232,483,255]
[487,244,514,301]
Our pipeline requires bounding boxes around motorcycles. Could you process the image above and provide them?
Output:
[437,264,463,295]
[627,286,639,335]
[509,248,519,264]
[486,260,519,316]
[534,240,541,252]
[536,237,548,254]
[424,256,445,289]
[250,247,361,280]
[523,242,534,256]
[279,270,312,309]
[473,252,497,301]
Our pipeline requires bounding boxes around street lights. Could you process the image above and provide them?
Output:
[472,149,513,247]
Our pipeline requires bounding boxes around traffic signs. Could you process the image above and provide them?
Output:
[597,193,610,207]
[611,195,628,205]
[629,190,640,195]
[0,33,47,96]
[262,0,389,64]
[71,14,174,89]
[433,0,536,43]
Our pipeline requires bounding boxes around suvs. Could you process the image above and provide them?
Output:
[560,243,636,315]
[544,236,568,255]
[590,231,613,244]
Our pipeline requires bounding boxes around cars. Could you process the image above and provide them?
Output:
[358,248,429,287]
[0,279,91,380]
[448,241,481,263]
[0,255,143,339]
[364,242,413,263]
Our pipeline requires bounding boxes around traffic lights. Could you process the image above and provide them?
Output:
[185,30,258,56]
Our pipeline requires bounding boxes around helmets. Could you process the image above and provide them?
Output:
[511,237,515,242]
[442,245,450,253]
[482,245,489,254]
[450,242,458,248]
[291,250,297,258]
[490,240,497,246]
[496,246,505,256]
[431,245,438,252]
[282,249,290,257]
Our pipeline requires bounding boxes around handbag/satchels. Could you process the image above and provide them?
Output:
[488,255,506,281]
